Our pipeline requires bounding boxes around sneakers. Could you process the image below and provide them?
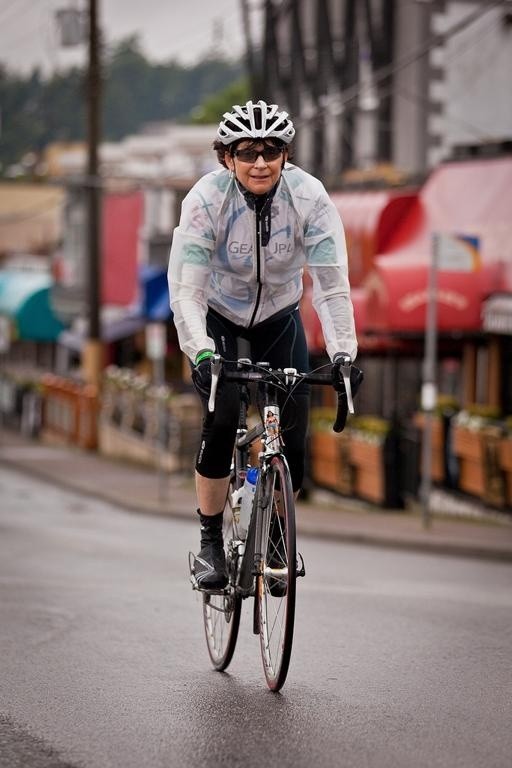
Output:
[266,544,290,598]
[193,543,229,588]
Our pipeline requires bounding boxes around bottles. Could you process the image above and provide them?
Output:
[236,466,260,543]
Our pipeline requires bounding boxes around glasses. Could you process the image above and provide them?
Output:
[225,146,286,163]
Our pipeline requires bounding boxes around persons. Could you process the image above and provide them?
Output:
[167,100,363,596]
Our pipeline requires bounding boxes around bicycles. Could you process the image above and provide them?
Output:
[189,354,362,696]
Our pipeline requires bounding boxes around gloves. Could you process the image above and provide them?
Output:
[190,358,226,401]
[330,356,365,397]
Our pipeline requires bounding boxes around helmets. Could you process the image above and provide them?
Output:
[214,98,297,149]
